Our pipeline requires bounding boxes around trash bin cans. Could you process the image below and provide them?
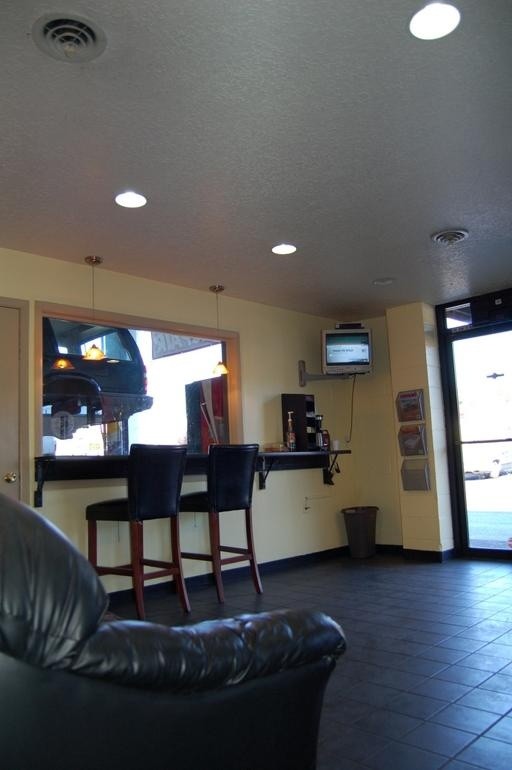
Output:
[341,506,380,558]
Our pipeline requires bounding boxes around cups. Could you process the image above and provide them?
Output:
[333,440,339,450]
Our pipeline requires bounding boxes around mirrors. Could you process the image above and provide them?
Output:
[34,299,243,459]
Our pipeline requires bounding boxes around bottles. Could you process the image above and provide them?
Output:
[316,430,330,447]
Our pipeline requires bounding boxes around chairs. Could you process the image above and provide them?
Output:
[180,443,263,604]
[0,490,349,770]
[86,445,191,621]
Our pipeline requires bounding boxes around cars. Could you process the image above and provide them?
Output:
[462,450,512,482]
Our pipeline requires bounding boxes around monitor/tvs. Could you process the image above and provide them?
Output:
[320,327,375,376]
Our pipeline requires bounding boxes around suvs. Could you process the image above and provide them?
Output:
[42,314,153,427]
